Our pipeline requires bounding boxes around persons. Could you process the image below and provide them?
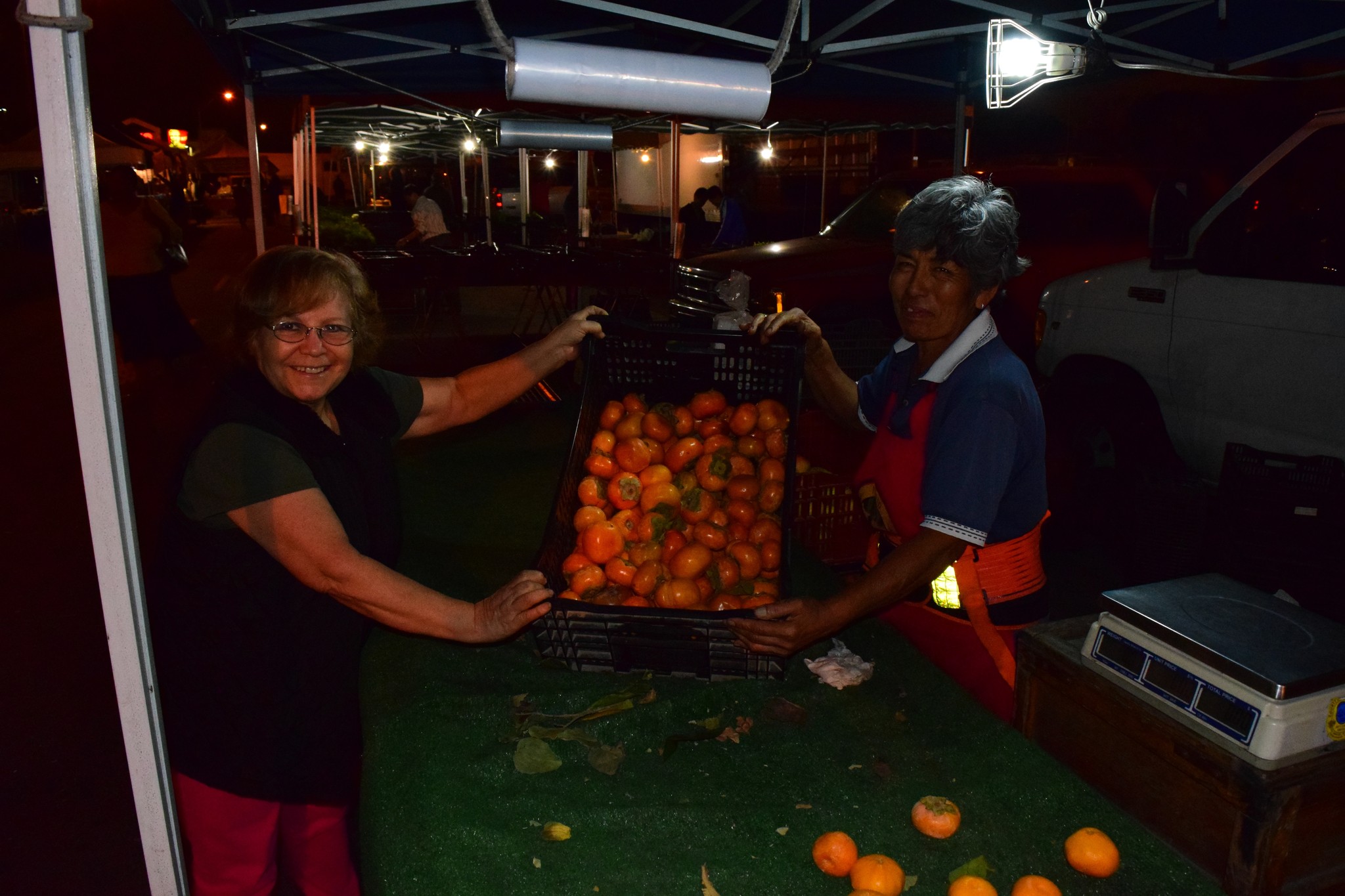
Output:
[396,187,452,248]
[676,187,708,260]
[102,164,201,408]
[173,244,607,896]
[168,164,446,226]
[729,175,1053,729]
[704,185,745,253]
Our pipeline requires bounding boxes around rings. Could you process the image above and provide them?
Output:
[762,314,767,323]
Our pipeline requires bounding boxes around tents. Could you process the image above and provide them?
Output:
[1,121,267,174]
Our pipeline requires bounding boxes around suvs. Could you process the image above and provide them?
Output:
[1034,108,1345,511]
[670,164,1158,381]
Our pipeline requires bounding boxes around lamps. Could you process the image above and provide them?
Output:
[979,14,1113,114]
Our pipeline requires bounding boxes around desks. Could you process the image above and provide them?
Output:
[352,436,1233,896]
[1013,618,1344,896]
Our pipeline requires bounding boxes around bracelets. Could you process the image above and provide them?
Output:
[405,237,409,242]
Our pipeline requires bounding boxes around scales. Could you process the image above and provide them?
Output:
[1081,573,1344,770]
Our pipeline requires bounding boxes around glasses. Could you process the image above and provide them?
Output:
[262,321,358,346]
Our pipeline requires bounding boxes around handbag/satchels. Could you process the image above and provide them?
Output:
[157,242,188,274]
